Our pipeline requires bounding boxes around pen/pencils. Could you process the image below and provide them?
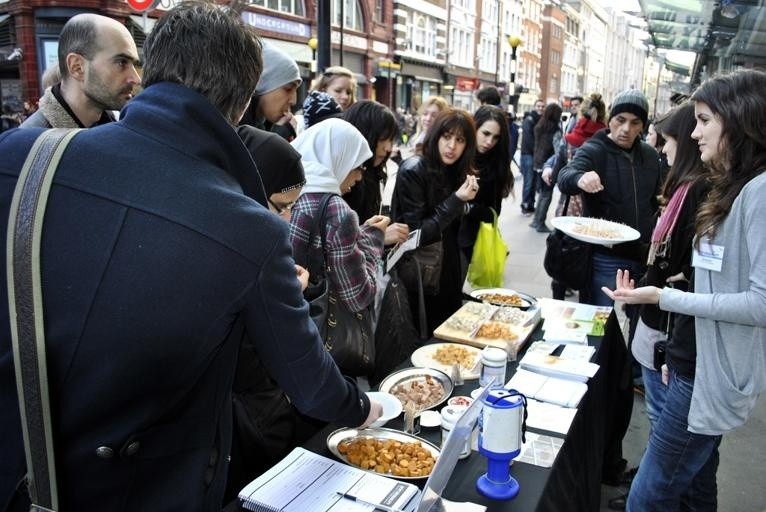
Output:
[336,492,403,512]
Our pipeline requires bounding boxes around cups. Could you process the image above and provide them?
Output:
[440,404,474,459]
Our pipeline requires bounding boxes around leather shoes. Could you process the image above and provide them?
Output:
[609,466,639,511]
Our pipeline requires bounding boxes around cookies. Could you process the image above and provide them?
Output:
[478,291,525,307]
[338,436,435,480]
[444,306,529,348]
[431,343,477,370]
[393,370,444,409]
[578,225,623,240]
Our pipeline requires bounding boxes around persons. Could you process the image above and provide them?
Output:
[520,91,670,483]
[601,69,766,511]
[228,48,520,500]
[0,1,382,511]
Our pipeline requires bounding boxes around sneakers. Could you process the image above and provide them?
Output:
[522,211,551,233]
[633,384,645,397]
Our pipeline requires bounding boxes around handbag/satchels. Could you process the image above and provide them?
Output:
[544,227,595,293]
[376,278,424,378]
[467,223,510,289]
[543,183,584,231]
[382,240,445,297]
[304,275,376,378]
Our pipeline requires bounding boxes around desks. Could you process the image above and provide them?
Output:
[221,297,639,512]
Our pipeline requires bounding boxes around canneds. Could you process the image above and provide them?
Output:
[441,395,475,460]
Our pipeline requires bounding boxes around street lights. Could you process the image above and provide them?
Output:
[507,37,521,117]
[309,38,318,76]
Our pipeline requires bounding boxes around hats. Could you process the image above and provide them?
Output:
[302,89,345,130]
[254,38,303,97]
[607,87,649,130]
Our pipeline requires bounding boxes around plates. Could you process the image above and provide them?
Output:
[447,396,474,406]
[410,342,489,383]
[468,286,538,310]
[325,426,445,481]
[376,365,454,417]
[550,215,641,248]
[420,410,441,428]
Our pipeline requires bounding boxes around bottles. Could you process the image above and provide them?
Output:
[403,399,421,437]
